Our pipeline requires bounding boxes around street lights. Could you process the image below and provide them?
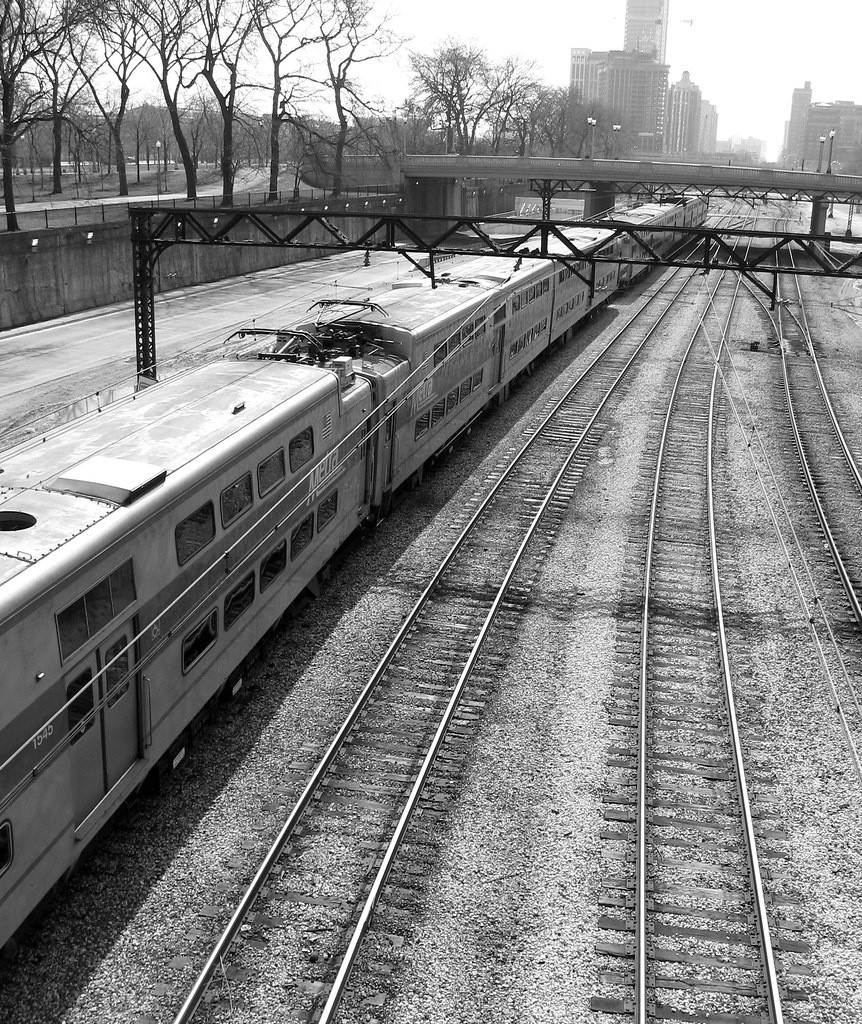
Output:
[403,115,408,156]
[155,140,163,194]
[612,123,621,160]
[587,116,596,160]
[826,128,835,174]
[450,120,456,153]
[817,134,826,173]
[444,120,449,155]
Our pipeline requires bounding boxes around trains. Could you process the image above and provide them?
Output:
[0,190,708,948]
